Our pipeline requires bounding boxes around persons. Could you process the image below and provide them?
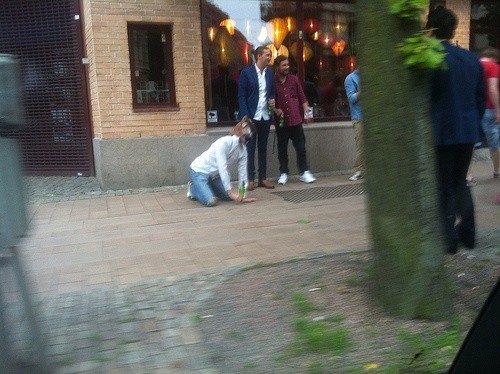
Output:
[187,117,259,207]
[424,9,489,254]
[344,64,366,181]
[237,47,277,190]
[273,55,316,185]
[466,48,500,186]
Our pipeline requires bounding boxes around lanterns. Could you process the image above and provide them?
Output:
[208,16,355,73]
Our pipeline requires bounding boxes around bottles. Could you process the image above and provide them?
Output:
[239,181,245,198]
[279,113,284,128]
[268,104,273,116]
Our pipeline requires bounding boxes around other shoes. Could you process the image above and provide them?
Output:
[247,181,254,191]
[257,179,275,188]
[494,173,500,177]
[186,181,192,199]
[349,172,363,181]
[465,178,477,186]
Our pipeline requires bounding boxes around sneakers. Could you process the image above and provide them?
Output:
[299,171,316,183]
[278,173,288,185]
